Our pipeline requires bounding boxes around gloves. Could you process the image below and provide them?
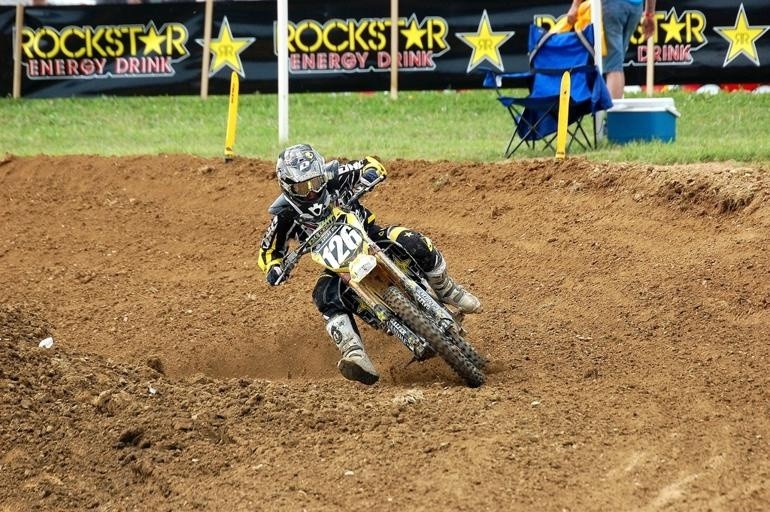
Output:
[357,169,378,192]
[266,265,288,286]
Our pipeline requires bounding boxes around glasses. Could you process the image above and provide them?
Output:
[278,173,328,198]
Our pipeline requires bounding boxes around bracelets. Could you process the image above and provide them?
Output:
[643,12,655,17]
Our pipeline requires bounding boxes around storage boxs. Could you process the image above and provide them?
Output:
[607,97,681,145]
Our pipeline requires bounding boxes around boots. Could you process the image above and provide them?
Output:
[425,257,480,314]
[326,311,379,385]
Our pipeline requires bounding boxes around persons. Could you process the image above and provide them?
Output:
[258,143,481,385]
[567,0,656,99]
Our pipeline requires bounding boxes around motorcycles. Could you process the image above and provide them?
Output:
[268,168,492,392]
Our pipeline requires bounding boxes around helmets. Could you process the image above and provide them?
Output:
[276,144,328,184]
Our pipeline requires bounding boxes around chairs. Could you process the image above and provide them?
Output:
[485,25,613,160]
